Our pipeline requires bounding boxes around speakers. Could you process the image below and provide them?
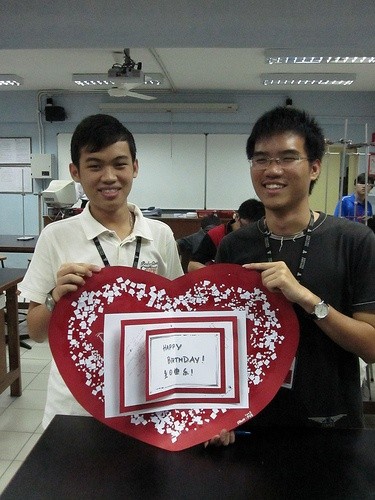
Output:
[45,106,66,121]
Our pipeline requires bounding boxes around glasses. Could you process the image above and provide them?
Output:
[237,217,248,228]
[247,153,314,171]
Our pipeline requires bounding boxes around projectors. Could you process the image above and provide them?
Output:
[107,68,145,83]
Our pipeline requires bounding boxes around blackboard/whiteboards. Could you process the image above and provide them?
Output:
[56,132,207,210]
[205,133,264,211]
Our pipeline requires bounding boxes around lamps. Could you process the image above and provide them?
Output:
[260,73,356,86]
[0,74,23,87]
[97,102,238,112]
[72,73,163,87]
[264,48,375,64]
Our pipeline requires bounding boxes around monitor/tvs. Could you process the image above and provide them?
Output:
[42,180,77,209]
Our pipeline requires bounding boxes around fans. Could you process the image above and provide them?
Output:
[72,76,156,100]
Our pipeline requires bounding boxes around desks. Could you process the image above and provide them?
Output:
[0,414,375,500]
[0,234,41,253]
[43,216,233,243]
[0,267,28,397]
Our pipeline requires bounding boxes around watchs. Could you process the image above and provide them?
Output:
[44,288,58,311]
[306,298,330,321]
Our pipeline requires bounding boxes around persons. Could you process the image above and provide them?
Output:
[203,107,375,449]
[177,200,266,272]
[335,173,374,225]
[23,115,183,430]
